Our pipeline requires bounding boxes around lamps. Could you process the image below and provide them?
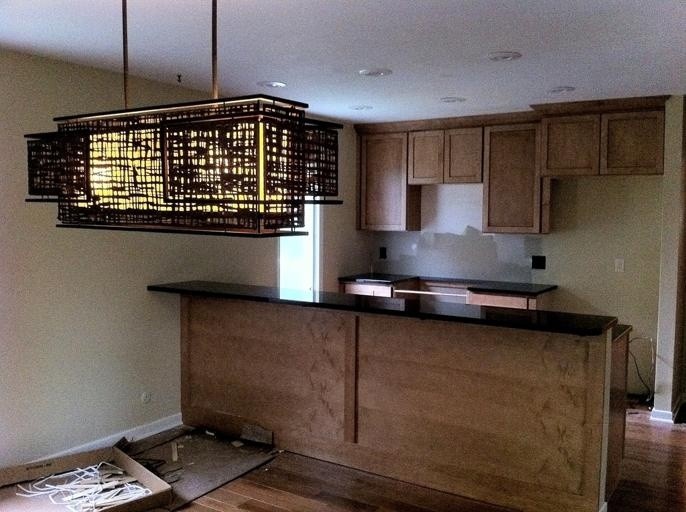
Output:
[23,0,346,239]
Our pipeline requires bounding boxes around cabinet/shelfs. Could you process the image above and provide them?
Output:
[408,115,483,186]
[528,94,669,179]
[484,113,542,236]
[354,121,409,235]
